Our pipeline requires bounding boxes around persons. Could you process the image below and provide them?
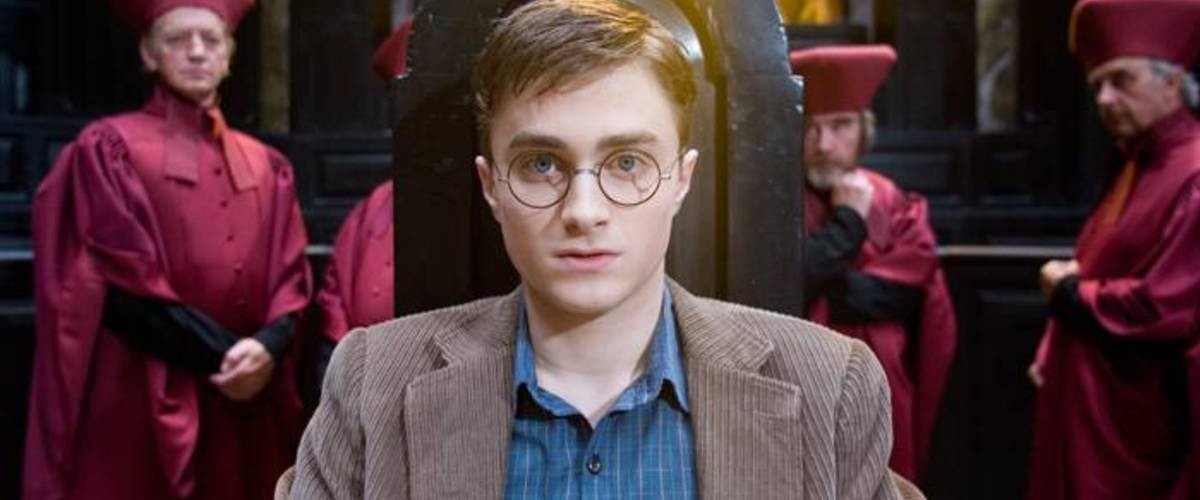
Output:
[270,0,929,500]
[306,19,410,387]
[788,47,959,485]
[1025,0,1200,500]
[22,0,317,500]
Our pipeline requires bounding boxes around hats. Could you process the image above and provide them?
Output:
[789,44,896,114]
[1068,0,1200,74]
[120,0,256,33]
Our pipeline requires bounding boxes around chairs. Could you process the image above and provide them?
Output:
[392,0,808,320]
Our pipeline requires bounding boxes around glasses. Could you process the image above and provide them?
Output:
[493,148,687,209]
